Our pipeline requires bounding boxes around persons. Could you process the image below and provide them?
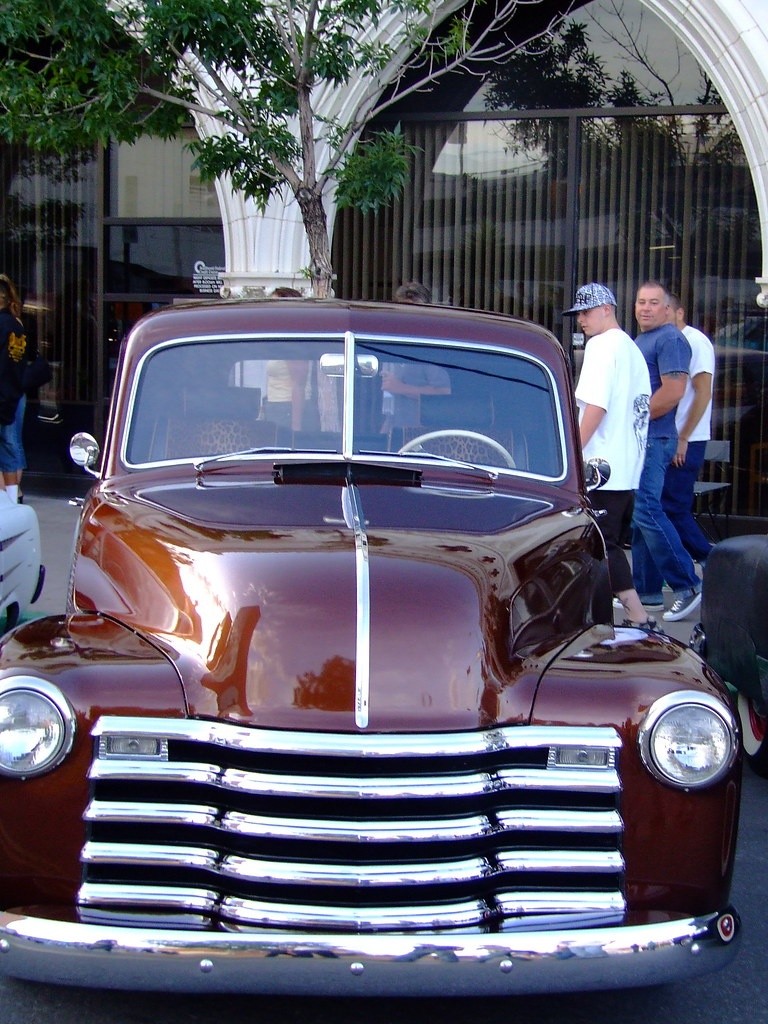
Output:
[266,286,313,431]
[379,282,451,435]
[48,282,108,477]
[0,275,29,504]
[613,280,715,622]
[562,283,658,634]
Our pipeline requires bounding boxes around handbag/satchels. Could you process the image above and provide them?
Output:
[35,351,54,386]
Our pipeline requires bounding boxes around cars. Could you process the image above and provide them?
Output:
[688,534,768,779]
[0,297,743,1001]
[0,489,47,637]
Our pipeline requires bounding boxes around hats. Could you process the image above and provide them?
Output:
[561,281,617,316]
[395,283,430,303]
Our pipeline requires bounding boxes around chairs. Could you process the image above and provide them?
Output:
[148,386,294,463]
[388,394,532,470]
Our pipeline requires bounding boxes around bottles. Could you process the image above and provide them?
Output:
[381,391,394,416]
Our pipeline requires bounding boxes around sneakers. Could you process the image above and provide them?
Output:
[621,615,664,635]
[662,591,702,621]
[612,598,664,611]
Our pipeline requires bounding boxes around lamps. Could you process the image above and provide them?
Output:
[648,220,682,260]
